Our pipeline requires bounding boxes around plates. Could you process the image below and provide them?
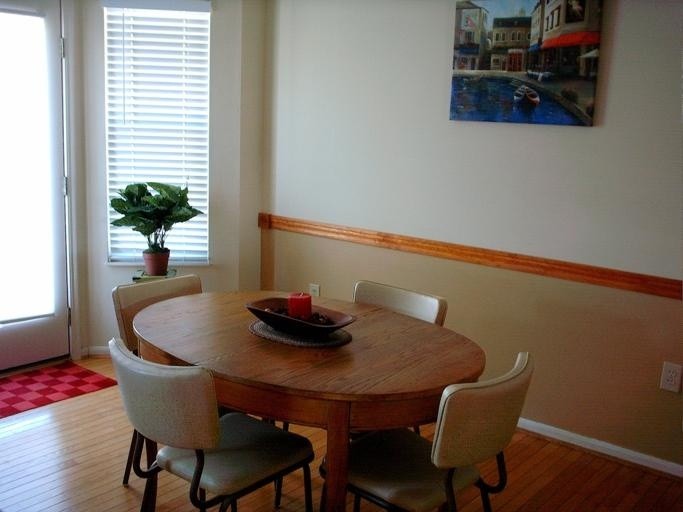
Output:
[244,297,358,341]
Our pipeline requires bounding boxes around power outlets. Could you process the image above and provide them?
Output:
[309,283,320,299]
[659,362,682,394]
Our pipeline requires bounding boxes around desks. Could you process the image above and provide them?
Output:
[134,291,486,512]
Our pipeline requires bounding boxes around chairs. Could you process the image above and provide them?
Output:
[112,275,205,485]
[319,352,535,511]
[350,281,450,436]
[109,337,314,512]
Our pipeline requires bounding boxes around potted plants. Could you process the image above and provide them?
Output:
[110,181,203,276]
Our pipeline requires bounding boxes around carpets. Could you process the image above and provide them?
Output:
[0,360,117,418]
